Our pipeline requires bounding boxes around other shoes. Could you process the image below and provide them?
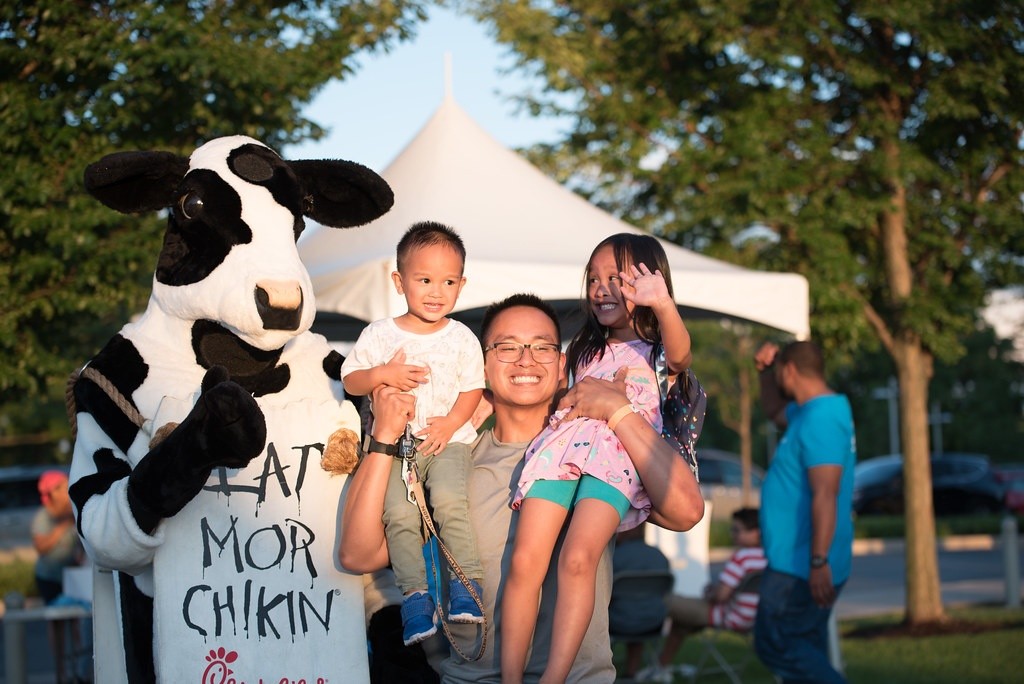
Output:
[636,664,673,683]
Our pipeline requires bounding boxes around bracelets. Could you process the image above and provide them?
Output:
[607,404,640,430]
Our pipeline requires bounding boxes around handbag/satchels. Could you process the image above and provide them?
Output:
[658,344,708,465]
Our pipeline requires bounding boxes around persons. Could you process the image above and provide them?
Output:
[338,292,704,684]
[752,341,858,684]
[498,234,691,684]
[339,221,486,645]
[29,471,85,605]
[610,507,769,684]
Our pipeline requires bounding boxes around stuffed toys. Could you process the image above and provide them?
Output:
[69,136,444,684]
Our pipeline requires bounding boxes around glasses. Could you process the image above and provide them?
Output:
[484,342,561,364]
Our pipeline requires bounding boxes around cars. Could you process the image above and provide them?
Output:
[693,449,769,497]
[852,451,1006,521]
[2,467,72,553]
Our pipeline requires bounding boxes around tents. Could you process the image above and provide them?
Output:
[295,77,844,679]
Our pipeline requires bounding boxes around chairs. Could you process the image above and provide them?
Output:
[602,570,671,684]
[683,570,772,683]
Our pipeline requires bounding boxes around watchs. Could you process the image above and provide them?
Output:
[363,433,398,456]
[811,556,827,567]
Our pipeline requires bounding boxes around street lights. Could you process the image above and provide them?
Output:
[878,387,900,458]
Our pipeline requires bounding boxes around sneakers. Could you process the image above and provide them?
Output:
[401,592,438,646]
[449,577,485,623]
